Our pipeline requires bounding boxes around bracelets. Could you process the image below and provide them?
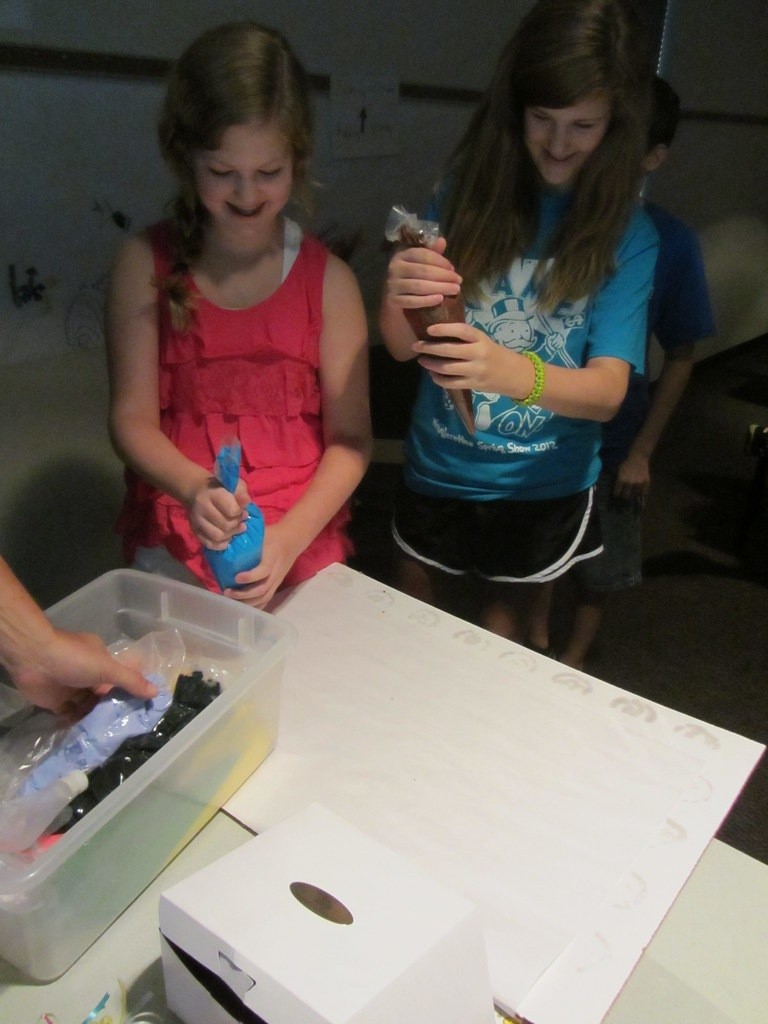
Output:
[509,351,544,406]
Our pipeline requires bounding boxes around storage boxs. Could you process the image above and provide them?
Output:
[0,566,289,980]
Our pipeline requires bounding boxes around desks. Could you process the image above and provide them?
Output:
[0,563,768,1024]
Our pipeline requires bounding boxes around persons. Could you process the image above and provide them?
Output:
[104,24,372,614]
[380,0,661,638]
[522,78,718,666]
[0,557,159,725]
